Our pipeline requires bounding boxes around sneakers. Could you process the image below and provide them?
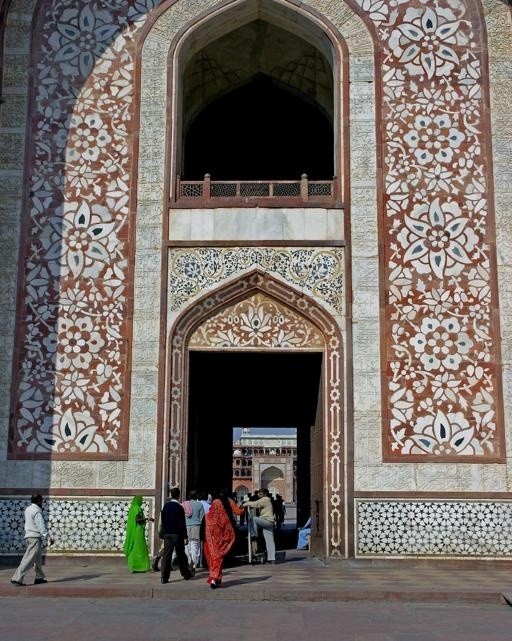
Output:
[185,574,194,579]
[34,578,46,583]
[161,577,168,583]
[10,580,25,585]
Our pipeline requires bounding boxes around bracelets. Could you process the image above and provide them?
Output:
[148,517,151,521]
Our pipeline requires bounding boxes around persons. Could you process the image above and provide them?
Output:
[123,495,155,574]
[152,487,287,589]
[10,493,48,586]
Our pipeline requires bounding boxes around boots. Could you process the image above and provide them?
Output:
[152,556,161,570]
